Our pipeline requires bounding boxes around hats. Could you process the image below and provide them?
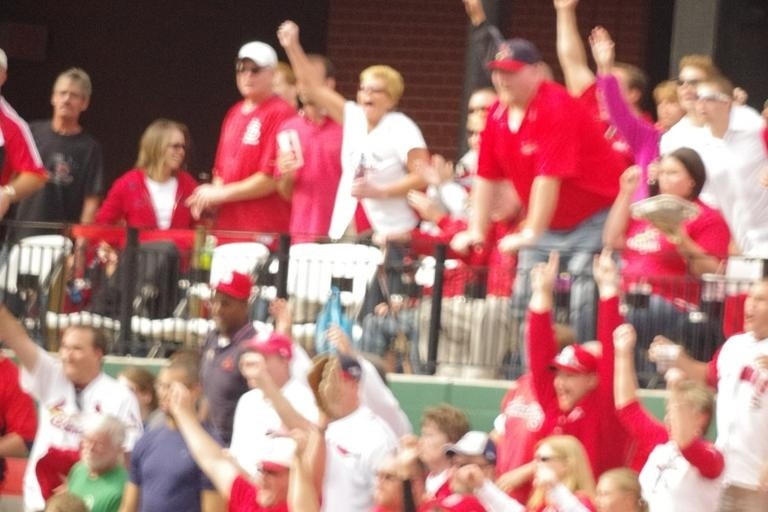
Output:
[484,36,542,73]
[548,343,600,375]
[237,40,280,69]
[215,270,253,299]
[339,352,363,383]
[243,332,293,360]
[440,430,498,466]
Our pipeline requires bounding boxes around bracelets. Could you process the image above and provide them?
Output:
[3,185,18,203]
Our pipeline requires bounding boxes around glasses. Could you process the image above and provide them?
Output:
[676,78,699,86]
[358,83,385,93]
[467,106,486,115]
[235,60,261,73]
[540,456,550,461]
[169,142,187,149]
[693,92,726,103]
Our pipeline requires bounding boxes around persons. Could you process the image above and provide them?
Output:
[407,82,520,299]
[468,1,554,90]
[194,242,314,448]
[323,320,415,439]
[412,109,520,222]
[0,300,145,511]
[419,430,498,512]
[8,68,103,247]
[399,404,472,512]
[116,368,159,434]
[271,54,343,245]
[96,120,213,230]
[271,61,298,109]
[117,363,226,512]
[231,331,320,476]
[555,1,652,166]
[368,446,403,512]
[0,352,39,482]
[451,434,596,511]
[519,246,628,485]
[436,0,767,250]
[606,143,738,389]
[647,281,767,511]
[661,52,722,156]
[0,48,48,218]
[488,374,544,505]
[67,415,130,511]
[529,463,648,512]
[684,76,767,259]
[589,25,685,205]
[159,380,321,511]
[450,39,629,383]
[185,41,301,248]
[277,19,430,241]
[238,349,400,512]
[613,323,726,512]
